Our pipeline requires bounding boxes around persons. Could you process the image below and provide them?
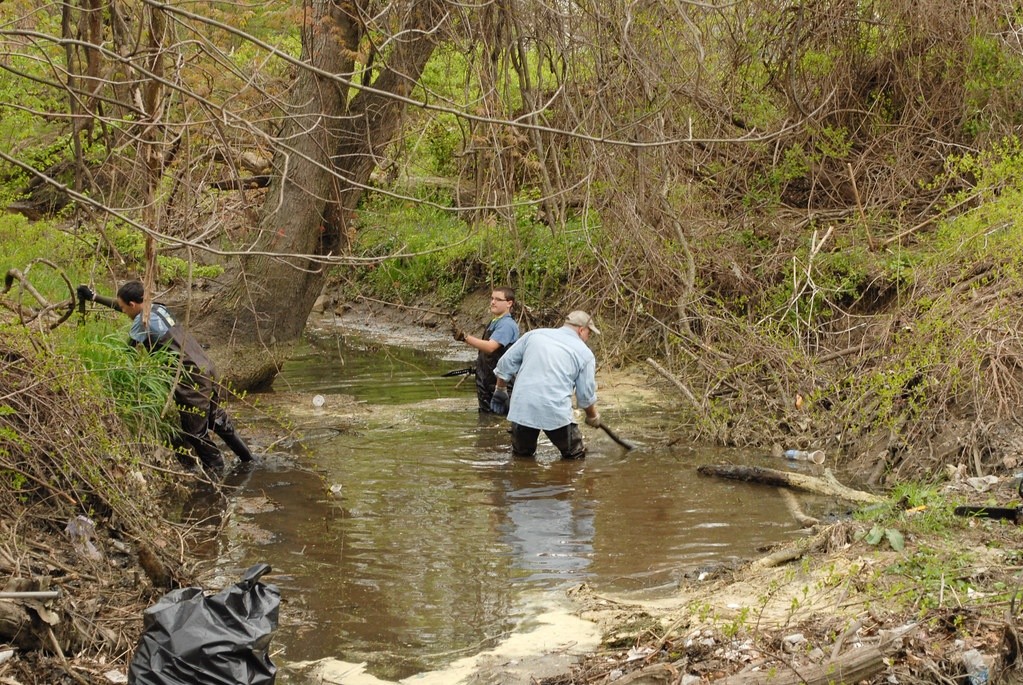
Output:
[450,286,521,415]
[490,310,602,460]
[74,280,255,471]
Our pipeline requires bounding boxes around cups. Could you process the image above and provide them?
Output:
[807,450,825,464]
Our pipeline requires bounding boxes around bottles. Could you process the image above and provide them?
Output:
[783,449,809,461]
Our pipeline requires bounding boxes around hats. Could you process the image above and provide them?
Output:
[565,310,601,334]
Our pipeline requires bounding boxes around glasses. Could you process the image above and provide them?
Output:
[489,297,508,301]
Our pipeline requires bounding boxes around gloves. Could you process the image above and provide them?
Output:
[448,317,460,333]
[586,412,601,429]
[76,283,96,301]
[452,329,468,342]
[489,384,509,414]
